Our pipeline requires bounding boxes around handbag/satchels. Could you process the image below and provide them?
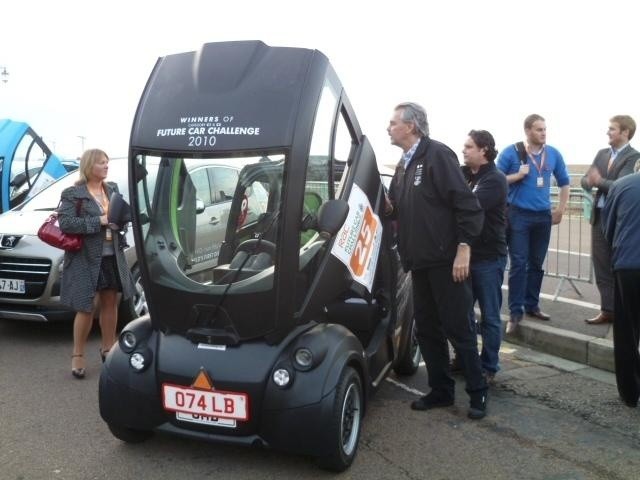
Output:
[38,214,83,254]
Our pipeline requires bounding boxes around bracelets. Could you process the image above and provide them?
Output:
[458,242,469,247]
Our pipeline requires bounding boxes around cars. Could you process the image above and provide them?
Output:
[0,116,79,212]
[0,157,280,327]
[99,40,421,456]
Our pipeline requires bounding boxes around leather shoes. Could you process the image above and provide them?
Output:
[73,349,109,382]
[585,311,615,325]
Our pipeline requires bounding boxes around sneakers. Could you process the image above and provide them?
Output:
[412,388,453,411]
[507,313,522,335]
[470,367,497,418]
[528,310,552,322]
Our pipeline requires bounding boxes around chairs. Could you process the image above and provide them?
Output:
[256,212,277,243]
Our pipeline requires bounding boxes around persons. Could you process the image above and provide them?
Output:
[496,114,570,334]
[448,130,509,384]
[387,103,489,418]
[601,158,640,408]
[58,149,128,379]
[580,114,640,323]
